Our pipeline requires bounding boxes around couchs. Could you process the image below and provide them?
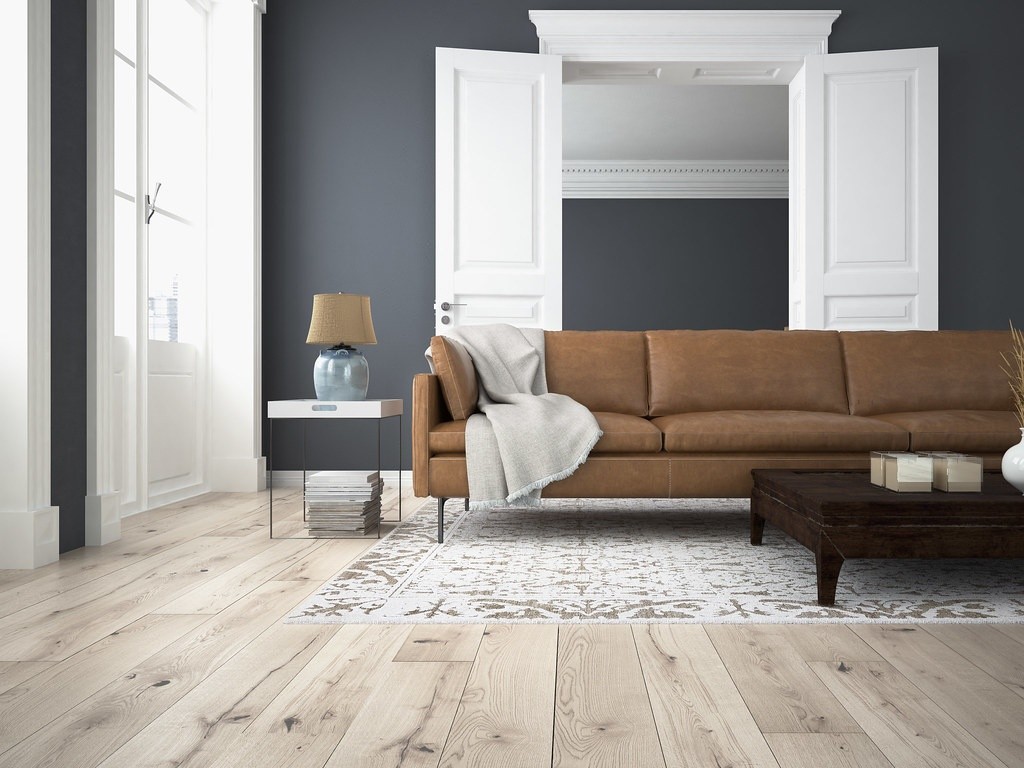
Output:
[413,330,1024,543]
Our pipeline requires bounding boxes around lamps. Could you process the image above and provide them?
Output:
[305,292,378,400]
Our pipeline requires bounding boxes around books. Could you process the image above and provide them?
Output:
[303,470,385,536]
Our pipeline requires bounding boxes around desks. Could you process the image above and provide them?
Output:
[268,398,403,540]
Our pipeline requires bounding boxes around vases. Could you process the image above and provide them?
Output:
[1001,428,1024,495]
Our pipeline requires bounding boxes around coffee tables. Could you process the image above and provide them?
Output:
[750,467,1024,606]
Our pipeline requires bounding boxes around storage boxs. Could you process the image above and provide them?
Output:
[917,451,983,492]
[871,451,914,488]
[885,455,932,493]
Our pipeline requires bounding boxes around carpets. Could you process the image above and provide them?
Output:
[292,495,1024,624]
